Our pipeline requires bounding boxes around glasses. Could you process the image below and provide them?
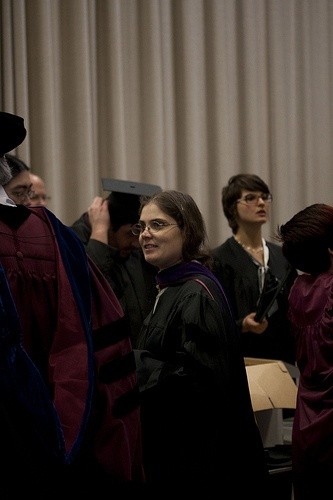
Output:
[130,222,179,236]
[9,190,35,202]
[31,195,52,202]
[237,193,273,204]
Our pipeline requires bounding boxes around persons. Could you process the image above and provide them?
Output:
[28,172,51,209]
[2,153,32,209]
[128,190,271,500]
[275,203,333,500]
[200,173,299,419]
[68,176,160,349]
[0,110,148,500]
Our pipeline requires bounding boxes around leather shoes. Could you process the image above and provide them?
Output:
[265,451,293,467]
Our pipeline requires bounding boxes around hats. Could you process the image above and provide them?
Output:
[101,175,163,220]
[0,112,27,155]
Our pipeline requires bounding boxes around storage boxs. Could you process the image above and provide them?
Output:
[245,356,298,413]
